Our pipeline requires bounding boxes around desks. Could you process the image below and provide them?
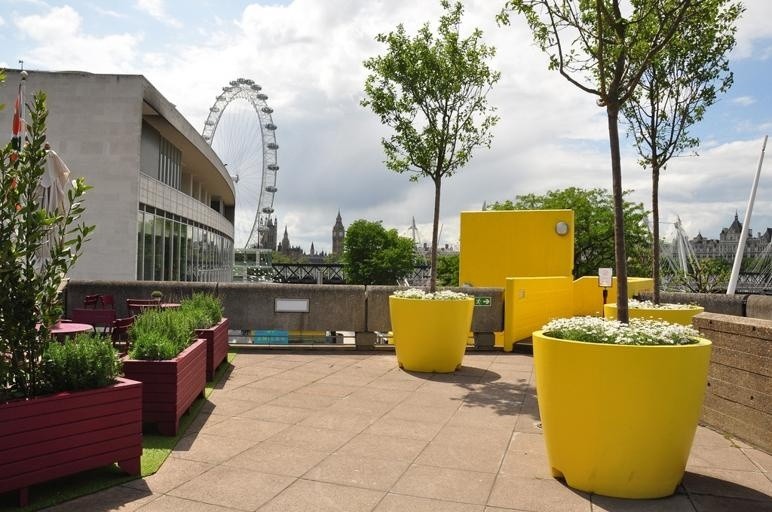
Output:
[43,317,71,343]
[129,303,181,313]
[34,323,93,342]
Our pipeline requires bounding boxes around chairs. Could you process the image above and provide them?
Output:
[72,308,115,339]
[100,294,116,336]
[84,294,99,326]
[112,315,137,358]
[127,298,162,314]
[111,321,134,358]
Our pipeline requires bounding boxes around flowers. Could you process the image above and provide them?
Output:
[627,298,694,310]
[542,315,701,344]
[392,287,466,300]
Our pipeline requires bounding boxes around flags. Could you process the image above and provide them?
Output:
[4,82,24,252]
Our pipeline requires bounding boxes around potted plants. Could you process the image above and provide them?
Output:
[119,307,207,438]
[496,0,746,499]
[359,0,501,373]
[152,291,162,301]
[0,91,142,505]
[179,290,230,382]
[574,0,745,327]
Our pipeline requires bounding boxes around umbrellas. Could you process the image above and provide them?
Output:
[19,140,72,331]
